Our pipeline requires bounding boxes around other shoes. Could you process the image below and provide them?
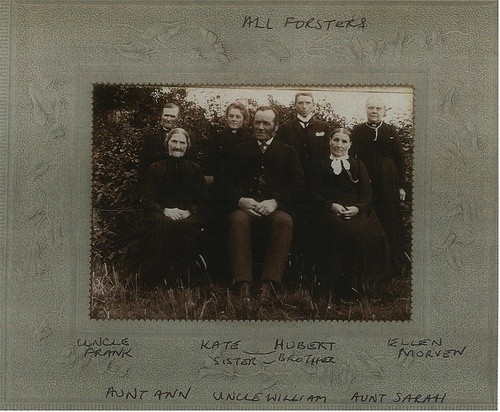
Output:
[239,282,252,302]
[258,281,272,305]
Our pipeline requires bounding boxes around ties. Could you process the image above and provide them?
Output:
[259,142,268,153]
[298,118,310,128]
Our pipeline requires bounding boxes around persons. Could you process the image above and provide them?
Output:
[309,128,389,302]
[140,129,207,289]
[201,102,254,194]
[138,103,179,177]
[276,93,332,281]
[350,95,406,276]
[217,107,306,310]
